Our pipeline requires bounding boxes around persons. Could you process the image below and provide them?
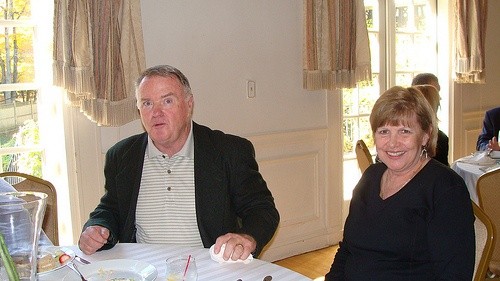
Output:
[325,72,475,281]
[77,64,280,262]
[476,107,500,155]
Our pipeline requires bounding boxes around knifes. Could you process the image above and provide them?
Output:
[74,254,91,265]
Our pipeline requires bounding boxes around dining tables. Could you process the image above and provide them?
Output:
[37,245,313,281]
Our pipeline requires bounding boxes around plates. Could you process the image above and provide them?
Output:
[36,244,75,276]
[63,259,157,281]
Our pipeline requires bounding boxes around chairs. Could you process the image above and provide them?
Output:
[477,168,500,277]
[0,171,59,245]
[472,202,495,281]
[355,140,373,172]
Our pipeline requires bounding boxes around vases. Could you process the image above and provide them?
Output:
[0,191,48,281]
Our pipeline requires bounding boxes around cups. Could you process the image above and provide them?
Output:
[165,255,198,281]
[0,191,49,280]
[491,150,500,159]
[474,151,487,162]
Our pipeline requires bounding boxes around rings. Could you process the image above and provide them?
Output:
[235,244,244,248]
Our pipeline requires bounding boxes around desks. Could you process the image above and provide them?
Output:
[451,151,500,205]
